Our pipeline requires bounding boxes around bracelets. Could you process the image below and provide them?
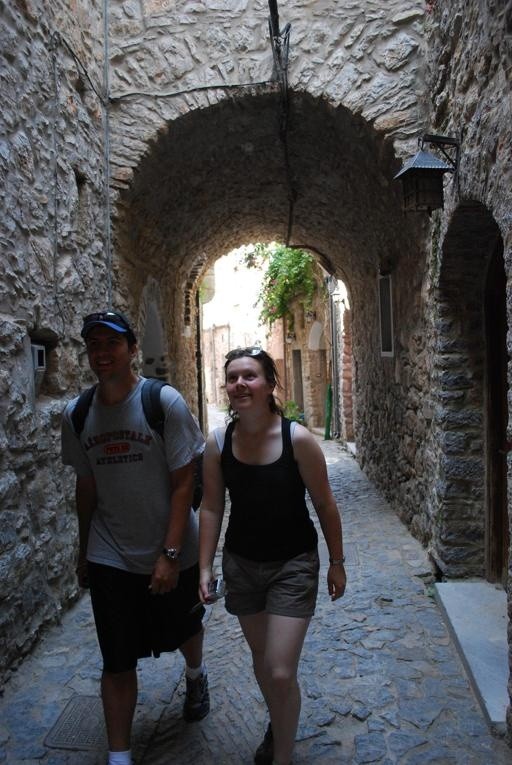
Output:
[329,558,344,566]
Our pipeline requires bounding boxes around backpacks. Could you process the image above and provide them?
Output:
[70,376,203,511]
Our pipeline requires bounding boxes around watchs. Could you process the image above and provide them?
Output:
[160,547,181,562]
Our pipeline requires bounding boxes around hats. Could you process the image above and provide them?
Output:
[81,310,137,345]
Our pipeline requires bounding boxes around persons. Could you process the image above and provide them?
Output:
[58,313,208,764]
[197,346,345,764]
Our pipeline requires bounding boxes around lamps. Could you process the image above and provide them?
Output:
[393,128,460,218]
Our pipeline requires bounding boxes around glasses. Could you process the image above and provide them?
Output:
[83,312,129,328]
[225,346,262,359]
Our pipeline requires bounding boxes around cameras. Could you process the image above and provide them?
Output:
[204,578,228,605]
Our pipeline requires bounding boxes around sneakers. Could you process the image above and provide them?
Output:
[183,659,210,723]
[255,721,274,765]
